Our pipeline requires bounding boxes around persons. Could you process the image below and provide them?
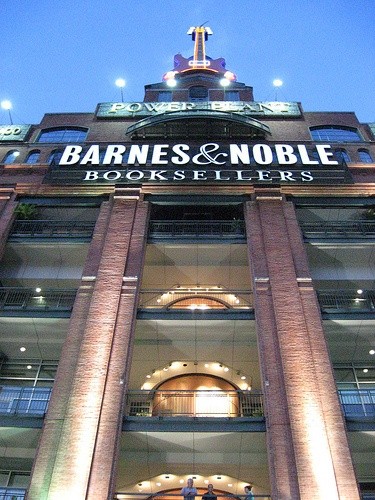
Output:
[202,483,217,500]
[180,479,197,500]
[244,486,254,500]
